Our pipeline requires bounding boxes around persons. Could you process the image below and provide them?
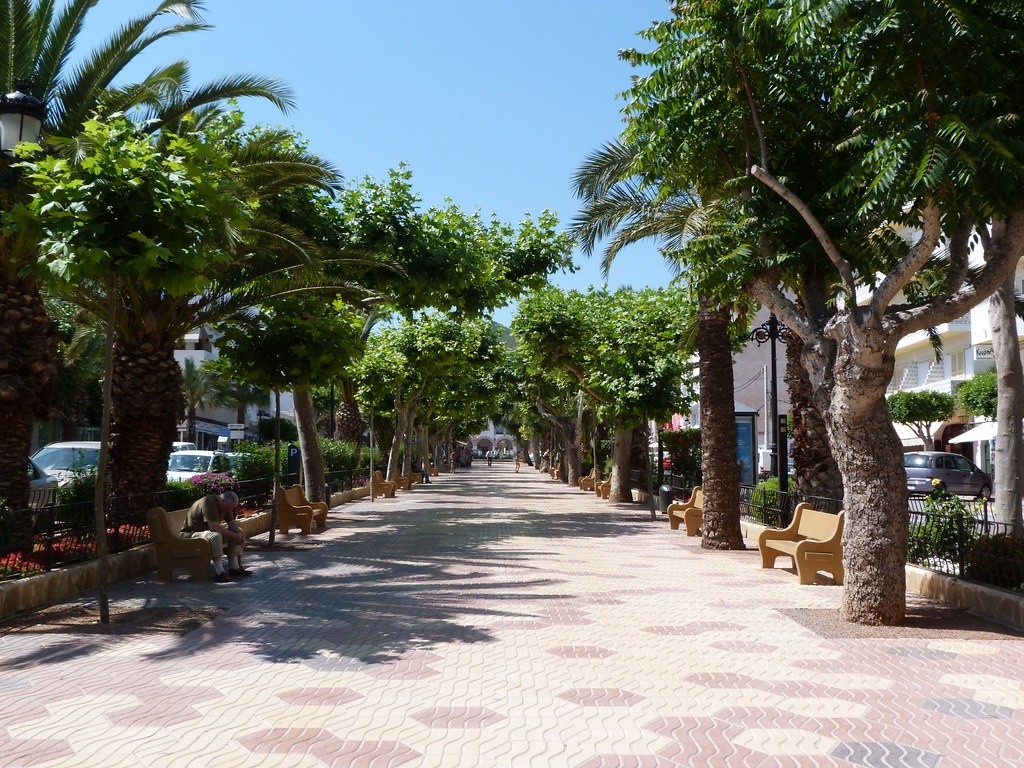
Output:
[450,449,458,474]
[179,491,253,584]
[513,451,521,473]
[411,461,432,484]
[485,449,493,466]
[540,449,551,474]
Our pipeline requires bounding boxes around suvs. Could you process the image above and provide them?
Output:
[31,440,116,495]
[165,449,229,485]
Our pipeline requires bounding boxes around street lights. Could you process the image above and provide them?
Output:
[737,311,800,527]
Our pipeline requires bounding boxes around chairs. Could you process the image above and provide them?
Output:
[945,460,954,469]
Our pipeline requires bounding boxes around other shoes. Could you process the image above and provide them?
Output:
[515,471,519,473]
[215,571,238,582]
[228,567,253,576]
[426,481,431,483]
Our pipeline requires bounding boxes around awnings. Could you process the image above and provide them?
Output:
[949,422,997,443]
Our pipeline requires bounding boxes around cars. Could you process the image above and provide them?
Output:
[905,451,991,499]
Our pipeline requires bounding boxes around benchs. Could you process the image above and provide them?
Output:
[372,470,398,498]
[549,462,561,479]
[409,463,423,484]
[395,468,411,490]
[147,507,228,584]
[666,485,704,537]
[277,484,329,535]
[596,472,612,499]
[578,468,601,491]
[759,502,846,586]
[428,462,438,476]
